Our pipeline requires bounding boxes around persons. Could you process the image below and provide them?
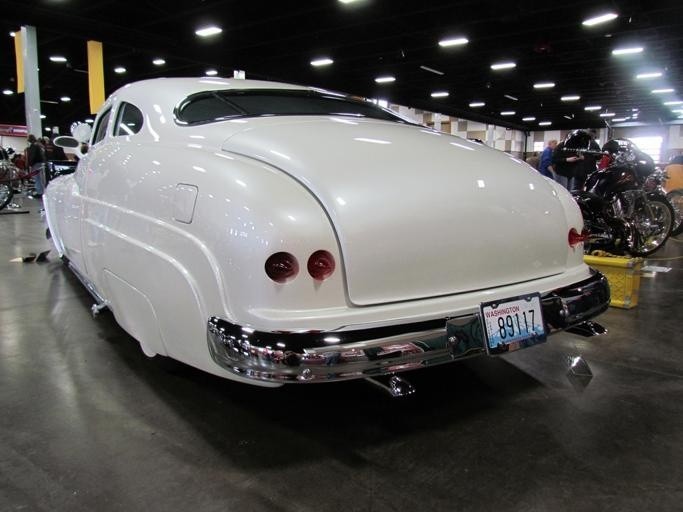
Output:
[24,134,47,197]
[552,130,584,191]
[538,139,560,180]
[584,128,603,173]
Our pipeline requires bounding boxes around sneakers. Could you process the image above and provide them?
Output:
[32,194,42,199]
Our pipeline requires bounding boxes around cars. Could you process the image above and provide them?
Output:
[42,75,610,398]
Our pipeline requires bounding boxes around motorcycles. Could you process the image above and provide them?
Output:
[0,147,18,211]
[562,137,683,256]
